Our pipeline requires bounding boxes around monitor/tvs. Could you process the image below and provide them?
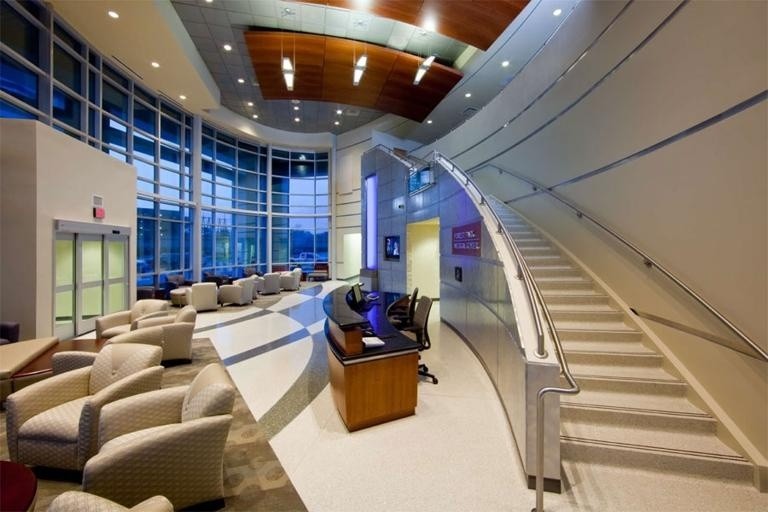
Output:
[352,283,363,312]
[385,236,400,258]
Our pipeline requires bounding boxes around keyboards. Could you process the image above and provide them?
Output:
[363,303,372,311]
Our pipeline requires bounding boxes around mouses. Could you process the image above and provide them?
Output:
[368,299,372,302]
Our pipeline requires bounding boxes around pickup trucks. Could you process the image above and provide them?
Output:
[287,251,327,270]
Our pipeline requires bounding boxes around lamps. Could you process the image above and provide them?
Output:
[352,21,368,85]
[280,8,296,91]
[413,32,436,85]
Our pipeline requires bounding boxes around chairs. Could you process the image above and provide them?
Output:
[390,288,418,327]
[394,296,438,384]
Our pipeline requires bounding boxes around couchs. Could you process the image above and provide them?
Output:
[94,297,197,364]
[136,263,302,312]
[5,343,237,511]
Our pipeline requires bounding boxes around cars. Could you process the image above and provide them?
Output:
[136,253,256,288]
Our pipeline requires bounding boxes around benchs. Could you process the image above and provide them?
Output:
[309,272,327,281]
